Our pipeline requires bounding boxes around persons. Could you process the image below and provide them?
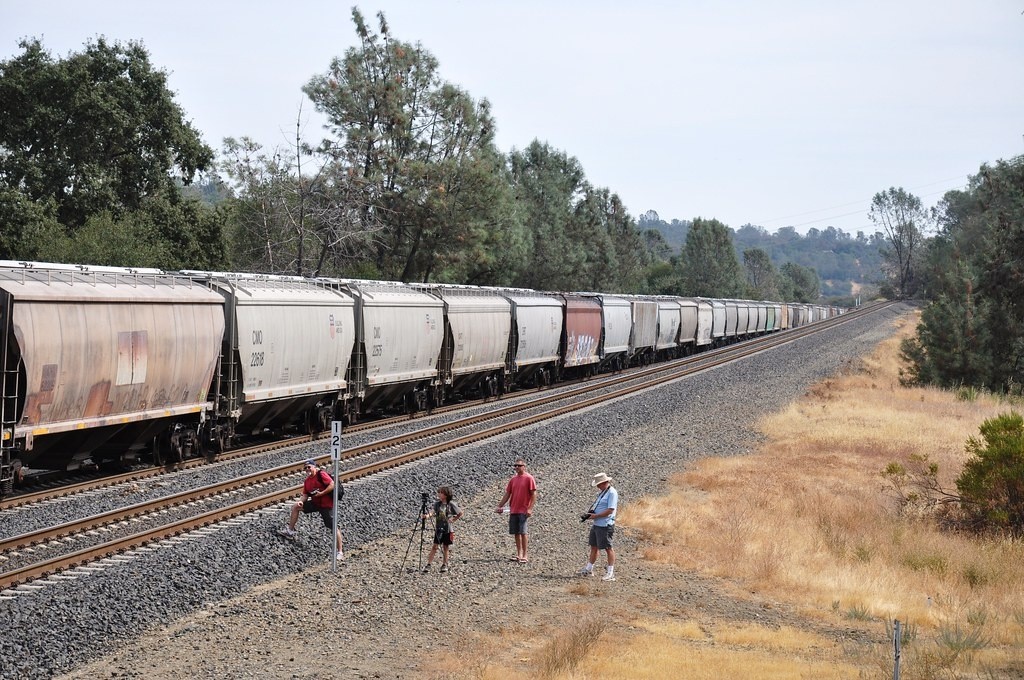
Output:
[575,472,618,582]
[496,459,537,563]
[420,486,463,572]
[280,460,343,560]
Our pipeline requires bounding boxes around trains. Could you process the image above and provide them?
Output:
[0,260,851,494]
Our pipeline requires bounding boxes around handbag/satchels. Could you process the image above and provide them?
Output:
[317,469,345,500]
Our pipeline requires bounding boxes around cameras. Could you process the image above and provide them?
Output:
[437,527,444,540]
[581,510,594,522]
[422,493,429,499]
[308,488,320,497]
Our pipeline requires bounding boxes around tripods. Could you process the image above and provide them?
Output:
[398,499,452,574]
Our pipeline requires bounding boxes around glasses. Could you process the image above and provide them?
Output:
[514,464,525,467]
[303,466,312,469]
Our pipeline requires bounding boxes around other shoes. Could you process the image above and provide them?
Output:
[280,530,293,539]
[424,565,432,572]
[510,555,521,561]
[601,572,615,581]
[575,567,594,577]
[336,552,344,560]
[519,558,528,563]
[441,564,448,571]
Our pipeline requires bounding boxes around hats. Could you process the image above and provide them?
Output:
[592,472,612,486]
[304,460,317,467]
